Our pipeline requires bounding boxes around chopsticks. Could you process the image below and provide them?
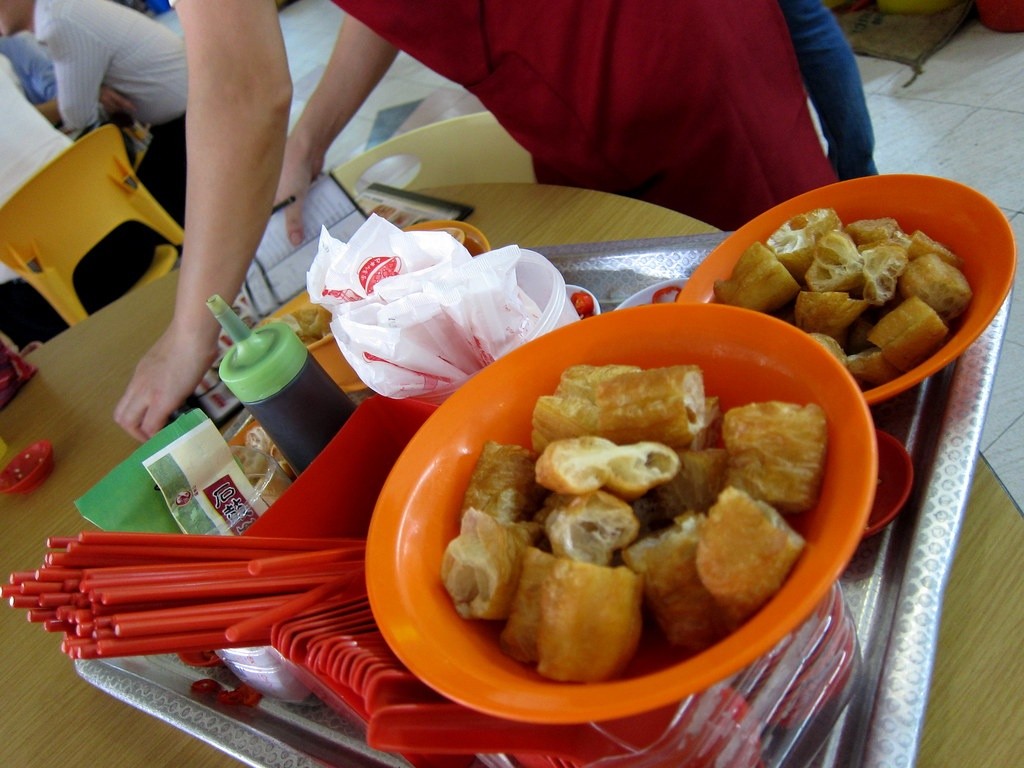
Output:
[0,531,366,660]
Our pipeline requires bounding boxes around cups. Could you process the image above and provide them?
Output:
[219,446,292,536]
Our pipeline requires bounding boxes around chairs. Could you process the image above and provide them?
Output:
[332,110,537,192]
[0,125,184,326]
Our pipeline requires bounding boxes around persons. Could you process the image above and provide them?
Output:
[114,0,877,443]
[0,0,189,356]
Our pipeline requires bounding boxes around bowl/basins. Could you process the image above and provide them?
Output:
[864,425,912,541]
[365,303,879,723]
[0,439,55,497]
[677,172,1015,404]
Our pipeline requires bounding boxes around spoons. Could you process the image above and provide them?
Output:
[272,584,854,768]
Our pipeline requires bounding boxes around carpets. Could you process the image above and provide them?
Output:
[829,0,973,88]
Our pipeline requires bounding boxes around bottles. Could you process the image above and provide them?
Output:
[206,293,360,477]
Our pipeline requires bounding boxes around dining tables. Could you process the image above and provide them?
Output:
[0,184,1023,767]
